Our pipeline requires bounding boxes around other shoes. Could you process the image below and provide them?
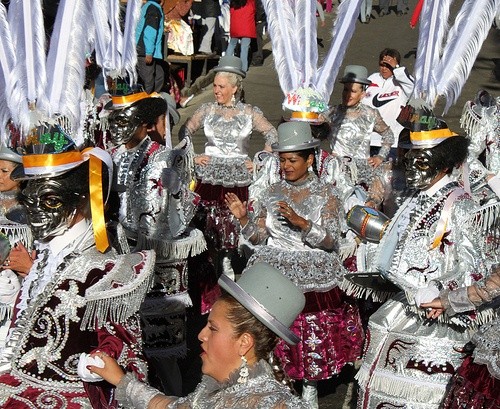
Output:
[182,94,194,107]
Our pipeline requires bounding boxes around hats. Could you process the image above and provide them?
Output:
[283,111,330,142]
[398,119,467,149]
[0,147,23,164]
[10,124,94,180]
[158,92,180,126]
[218,262,306,346]
[104,84,167,116]
[338,65,371,85]
[271,121,321,152]
[213,56,246,79]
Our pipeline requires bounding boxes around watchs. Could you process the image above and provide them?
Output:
[394,64,400,69]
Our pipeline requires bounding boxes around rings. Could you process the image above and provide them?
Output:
[7,257,11,262]
[101,351,107,359]
[286,211,290,215]
[287,204,290,208]
[232,199,235,202]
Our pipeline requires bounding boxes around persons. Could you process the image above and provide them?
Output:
[0,0,499,409]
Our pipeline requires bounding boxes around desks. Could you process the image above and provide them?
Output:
[165,52,220,89]
